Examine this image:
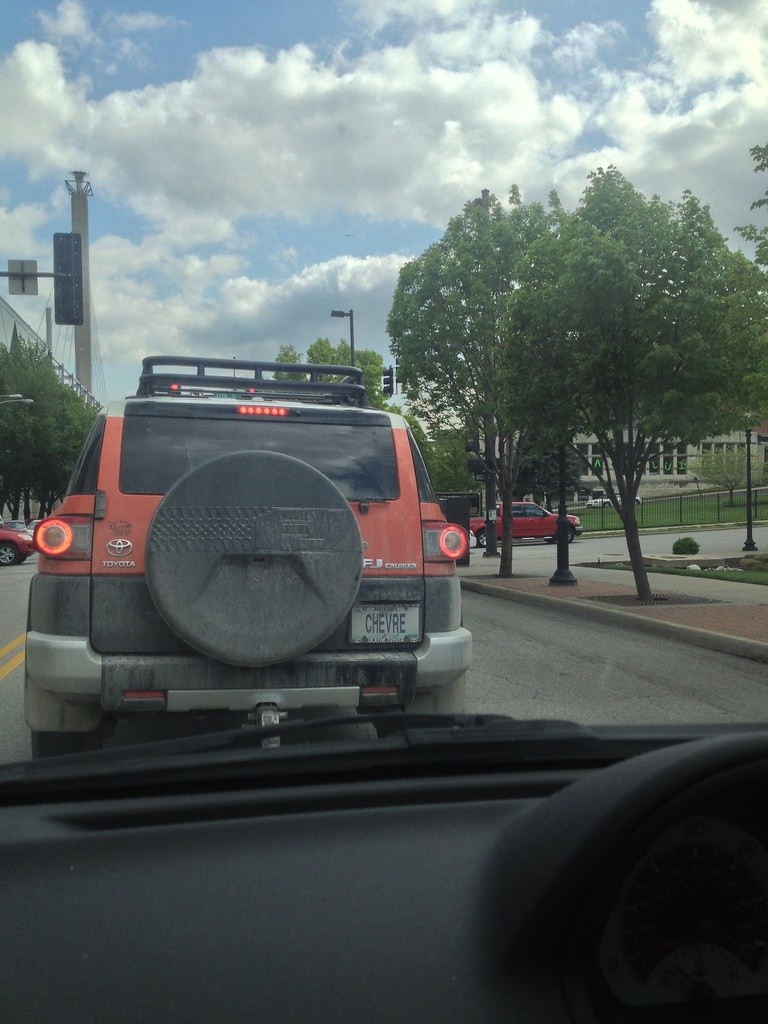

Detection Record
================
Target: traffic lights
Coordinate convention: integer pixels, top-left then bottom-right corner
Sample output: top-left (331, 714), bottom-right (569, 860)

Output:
top-left (396, 356), bottom-right (408, 383)
top-left (382, 365), bottom-right (394, 397)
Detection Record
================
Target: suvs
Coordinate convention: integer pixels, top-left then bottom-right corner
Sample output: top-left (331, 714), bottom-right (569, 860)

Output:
top-left (22, 354), bottom-right (474, 761)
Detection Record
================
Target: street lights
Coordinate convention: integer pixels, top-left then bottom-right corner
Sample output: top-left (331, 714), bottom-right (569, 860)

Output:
top-left (330, 308), bottom-right (355, 367)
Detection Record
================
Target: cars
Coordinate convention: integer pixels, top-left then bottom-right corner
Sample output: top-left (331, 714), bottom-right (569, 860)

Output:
top-left (586, 492), bottom-right (642, 509)
top-left (0, 518), bottom-right (42, 567)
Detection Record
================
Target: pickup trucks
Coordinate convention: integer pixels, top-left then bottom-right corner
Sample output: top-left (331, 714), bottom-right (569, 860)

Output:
top-left (469, 500), bottom-right (582, 548)
